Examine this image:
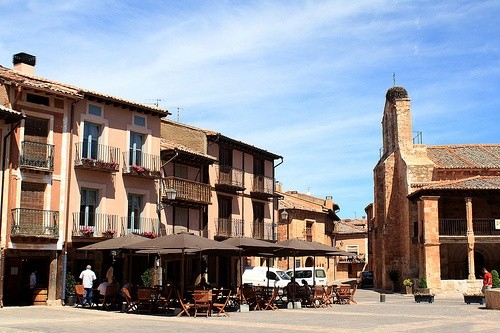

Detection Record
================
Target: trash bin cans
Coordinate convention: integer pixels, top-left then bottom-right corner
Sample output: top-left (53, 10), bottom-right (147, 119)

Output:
top-left (362, 271), bottom-right (374, 288)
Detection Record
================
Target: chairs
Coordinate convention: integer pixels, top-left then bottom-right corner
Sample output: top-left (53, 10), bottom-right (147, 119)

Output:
top-left (76, 284), bottom-right (358, 318)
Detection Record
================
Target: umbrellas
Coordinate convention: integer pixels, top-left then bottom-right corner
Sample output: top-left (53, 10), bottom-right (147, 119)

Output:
top-left (123, 229), bottom-right (242, 300)
top-left (289, 246), bottom-right (353, 284)
top-left (199, 236), bottom-right (284, 283)
top-left (76, 233), bottom-right (151, 251)
top-left (271, 238), bottom-right (341, 283)
top-left (135, 246), bottom-right (199, 254)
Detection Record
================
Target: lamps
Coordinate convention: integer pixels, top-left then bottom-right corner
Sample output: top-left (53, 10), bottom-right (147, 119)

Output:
top-left (277, 209), bottom-right (288, 225)
top-left (158, 186), bottom-right (176, 211)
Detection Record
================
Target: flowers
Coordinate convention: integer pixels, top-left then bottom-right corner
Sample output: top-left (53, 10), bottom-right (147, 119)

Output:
top-left (145, 231), bottom-right (157, 239)
top-left (79, 227), bottom-right (94, 233)
top-left (85, 157), bottom-right (117, 169)
top-left (403, 278), bottom-right (413, 286)
top-left (133, 165), bottom-right (161, 176)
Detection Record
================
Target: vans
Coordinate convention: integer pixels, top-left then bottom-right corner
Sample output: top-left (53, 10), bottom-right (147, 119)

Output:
top-left (280, 265), bottom-right (327, 289)
top-left (358, 270), bottom-right (374, 289)
top-left (241, 266), bottom-right (292, 296)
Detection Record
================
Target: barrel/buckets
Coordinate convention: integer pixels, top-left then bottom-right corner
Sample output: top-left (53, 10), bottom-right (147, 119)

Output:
top-left (361, 271), bottom-right (374, 290)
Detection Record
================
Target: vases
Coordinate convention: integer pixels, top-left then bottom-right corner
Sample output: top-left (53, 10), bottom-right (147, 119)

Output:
top-left (406, 286), bottom-right (413, 295)
top-left (130, 167), bottom-right (160, 179)
top-left (82, 230), bottom-right (94, 237)
top-left (82, 159), bottom-right (115, 170)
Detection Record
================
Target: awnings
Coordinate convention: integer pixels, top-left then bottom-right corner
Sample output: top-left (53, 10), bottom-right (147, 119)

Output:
top-left (0, 104), bottom-right (26, 137)
top-left (12, 80), bottom-right (84, 106)
top-left (160, 148), bottom-right (217, 168)
top-left (206, 131), bottom-right (284, 168)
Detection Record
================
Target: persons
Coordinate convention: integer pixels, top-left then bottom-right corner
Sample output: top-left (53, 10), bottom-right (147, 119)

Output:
top-left (481, 267), bottom-right (493, 292)
top-left (119, 279), bottom-right (132, 296)
top-left (79, 265), bottom-right (97, 307)
top-left (302, 280), bottom-right (312, 295)
top-left (95, 277), bottom-right (110, 306)
top-left (286, 277), bottom-right (300, 299)
top-left (111, 276), bottom-right (120, 292)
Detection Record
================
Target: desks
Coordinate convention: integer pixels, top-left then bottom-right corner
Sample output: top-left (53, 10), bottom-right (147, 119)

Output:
top-left (192, 293), bottom-right (216, 316)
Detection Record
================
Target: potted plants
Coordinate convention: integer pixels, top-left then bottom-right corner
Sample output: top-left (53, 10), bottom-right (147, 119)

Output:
top-left (385, 267), bottom-right (402, 292)
top-left (65, 272), bottom-right (81, 305)
top-left (418, 278), bottom-right (431, 294)
top-left (137, 268), bottom-right (156, 303)
top-left (461, 290), bottom-right (485, 305)
top-left (414, 293), bottom-right (435, 304)
